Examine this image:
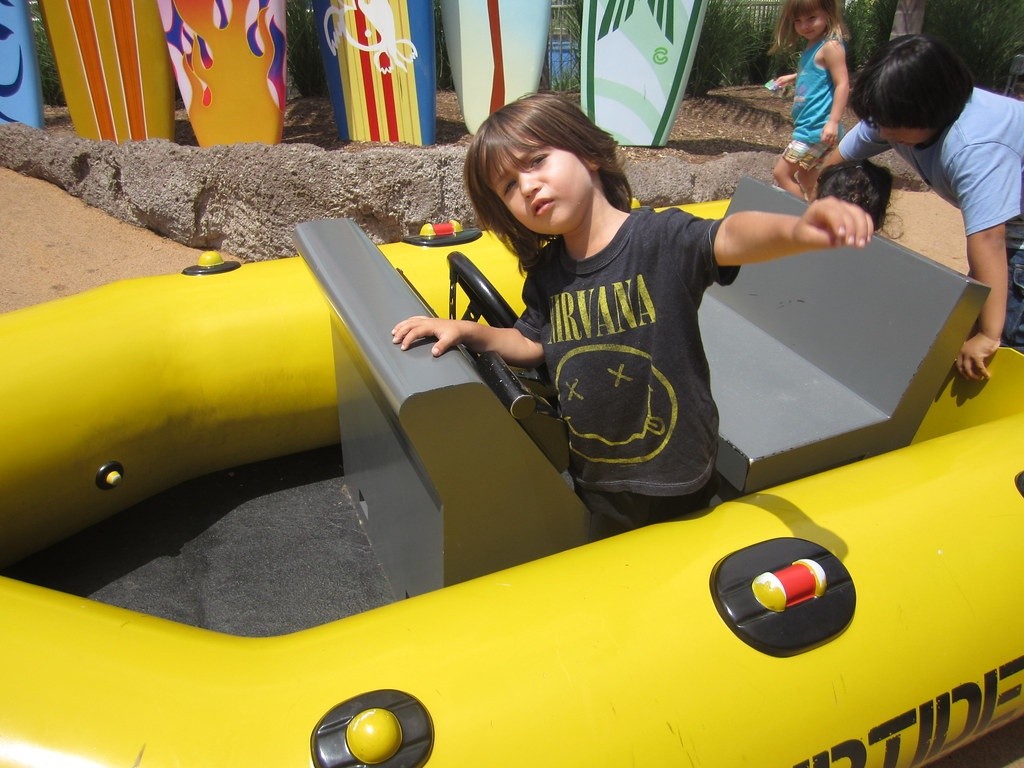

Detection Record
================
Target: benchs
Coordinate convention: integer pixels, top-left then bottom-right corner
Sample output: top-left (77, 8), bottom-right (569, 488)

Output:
top-left (698, 175), bottom-right (991, 503)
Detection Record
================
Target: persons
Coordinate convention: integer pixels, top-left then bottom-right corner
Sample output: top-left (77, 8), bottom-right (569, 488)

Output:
top-left (390, 84), bottom-right (873, 527)
top-left (806, 34), bottom-right (1024, 383)
top-left (772, 0), bottom-right (849, 198)
top-left (816, 158), bottom-right (893, 233)
